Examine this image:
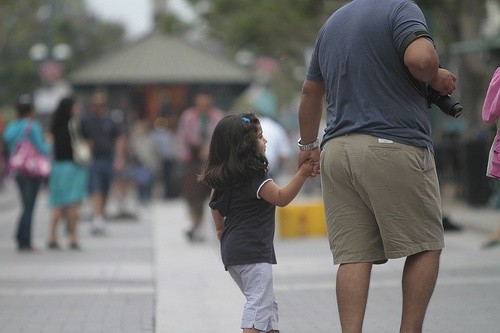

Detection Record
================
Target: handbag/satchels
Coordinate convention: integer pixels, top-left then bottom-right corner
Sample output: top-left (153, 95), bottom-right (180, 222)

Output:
top-left (8, 139), bottom-right (50, 179)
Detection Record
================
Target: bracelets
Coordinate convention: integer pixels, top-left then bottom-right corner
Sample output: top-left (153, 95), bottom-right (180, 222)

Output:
top-left (297, 137), bottom-right (320, 150)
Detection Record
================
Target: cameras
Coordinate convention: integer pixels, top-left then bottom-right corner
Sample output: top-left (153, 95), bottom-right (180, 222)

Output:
top-left (427, 84), bottom-right (464, 118)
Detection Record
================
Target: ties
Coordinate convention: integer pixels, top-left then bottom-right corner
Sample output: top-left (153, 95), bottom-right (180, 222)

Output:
top-left (199, 114), bottom-right (208, 141)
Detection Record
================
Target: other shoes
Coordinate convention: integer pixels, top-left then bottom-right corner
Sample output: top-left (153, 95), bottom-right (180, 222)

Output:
top-left (17, 245), bottom-right (39, 255)
top-left (442, 217), bottom-right (462, 231)
top-left (90, 222), bottom-right (107, 236)
top-left (113, 211), bottom-right (138, 221)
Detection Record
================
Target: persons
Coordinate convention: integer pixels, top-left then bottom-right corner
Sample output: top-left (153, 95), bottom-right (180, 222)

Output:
top-left (482, 66), bottom-right (500, 182)
top-left (177, 92), bottom-right (293, 242)
top-left (197, 112), bottom-right (319, 333)
top-left (0, 89), bottom-right (125, 252)
top-left (298, 0), bottom-right (457, 333)
top-left (120, 117), bottom-right (182, 207)
